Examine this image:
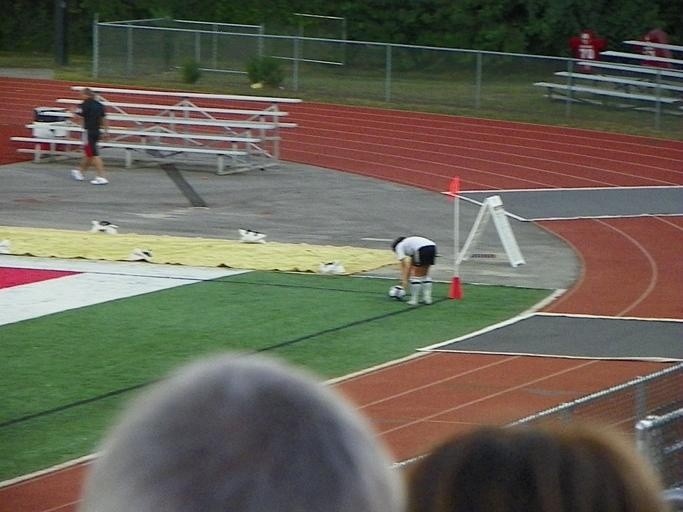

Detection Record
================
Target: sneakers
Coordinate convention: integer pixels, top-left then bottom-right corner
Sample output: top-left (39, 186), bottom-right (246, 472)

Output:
top-left (90, 176), bottom-right (108, 185)
top-left (72, 169), bottom-right (84, 180)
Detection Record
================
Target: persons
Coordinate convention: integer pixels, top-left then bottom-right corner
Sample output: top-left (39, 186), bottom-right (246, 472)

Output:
top-left (392, 236), bottom-right (436, 305)
top-left (71, 88), bottom-right (108, 185)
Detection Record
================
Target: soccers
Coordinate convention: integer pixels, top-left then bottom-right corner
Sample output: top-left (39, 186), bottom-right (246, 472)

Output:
top-left (389, 284), bottom-right (407, 301)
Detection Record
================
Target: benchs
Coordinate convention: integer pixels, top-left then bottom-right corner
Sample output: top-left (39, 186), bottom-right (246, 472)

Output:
top-left (533, 40), bottom-right (683, 128)
top-left (11, 86), bottom-right (302, 175)
